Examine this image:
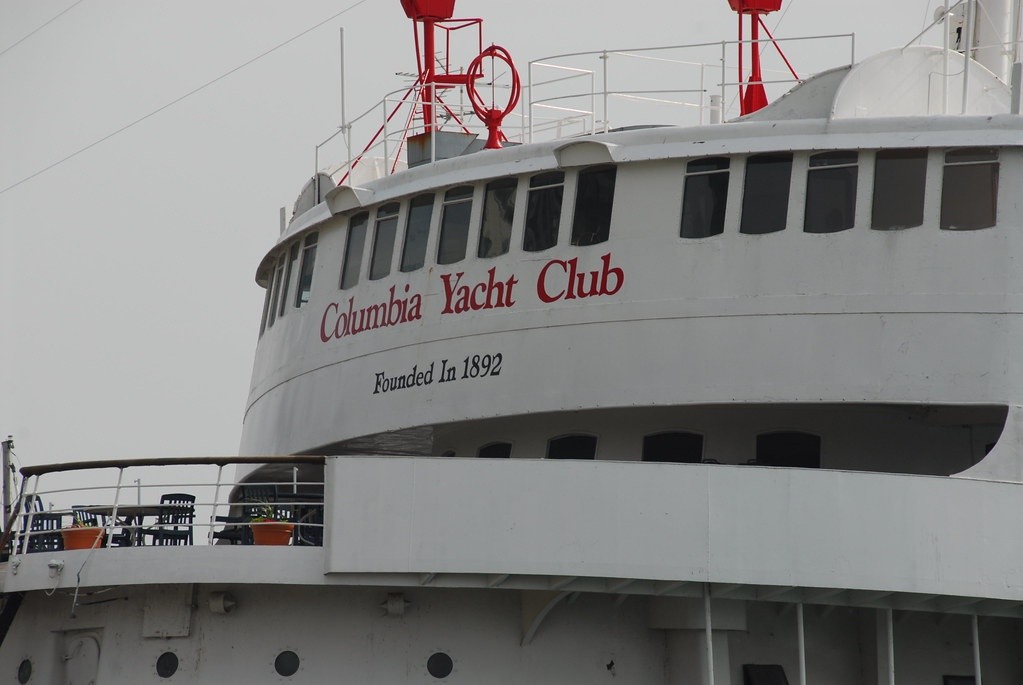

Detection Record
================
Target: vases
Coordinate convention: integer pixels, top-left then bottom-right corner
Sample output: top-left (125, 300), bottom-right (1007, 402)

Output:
top-left (251, 524), bottom-right (295, 545)
top-left (62, 526), bottom-right (105, 550)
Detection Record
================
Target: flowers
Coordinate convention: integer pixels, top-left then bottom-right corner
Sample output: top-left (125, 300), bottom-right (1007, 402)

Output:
top-left (247, 498), bottom-right (289, 524)
top-left (61, 516), bottom-right (93, 528)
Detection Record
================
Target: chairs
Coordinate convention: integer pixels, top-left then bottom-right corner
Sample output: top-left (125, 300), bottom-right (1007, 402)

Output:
top-left (71, 505), bottom-right (98, 528)
top-left (141, 493), bottom-right (196, 546)
top-left (85, 503), bottom-right (177, 547)
top-left (102, 514), bottom-right (135, 547)
top-left (25, 494), bottom-right (44, 513)
top-left (208, 501), bottom-right (254, 545)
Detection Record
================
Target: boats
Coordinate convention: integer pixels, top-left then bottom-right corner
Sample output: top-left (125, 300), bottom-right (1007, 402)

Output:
top-left (1, 0), bottom-right (1023, 685)
